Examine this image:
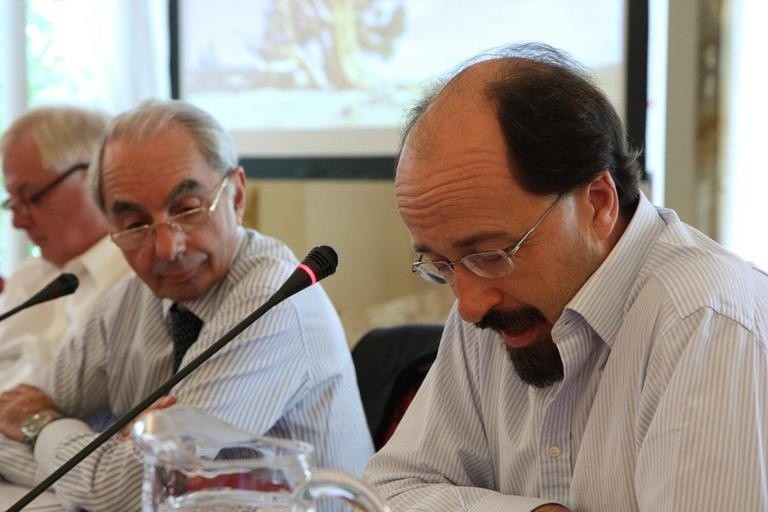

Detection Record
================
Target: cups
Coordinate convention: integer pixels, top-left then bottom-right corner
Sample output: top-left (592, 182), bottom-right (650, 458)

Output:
top-left (131, 404), bottom-right (391, 512)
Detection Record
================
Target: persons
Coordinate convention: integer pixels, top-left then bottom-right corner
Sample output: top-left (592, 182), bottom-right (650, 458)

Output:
top-left (1, 97), bottom-right (377, 511)
top-left (309, 41), bottom-right (768, 512)
top-left (0, 103), bottom-right (138, 396)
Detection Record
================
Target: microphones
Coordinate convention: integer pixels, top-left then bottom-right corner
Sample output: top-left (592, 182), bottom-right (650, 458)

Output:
top-left (4, 244), bottom-right (338, 512)
top-left (0, 273), bottom-right (78, 321)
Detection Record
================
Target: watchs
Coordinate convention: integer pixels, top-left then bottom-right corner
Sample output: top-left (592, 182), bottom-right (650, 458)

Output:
top-left (21, 407), bottom-right (63, 450)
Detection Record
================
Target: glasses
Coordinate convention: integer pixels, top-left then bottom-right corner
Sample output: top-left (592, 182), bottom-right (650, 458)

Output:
top-left (0, 161), bottom-right (89, 216)
top-left (411, 189), bottom-right (567, 285)
top-left (108, 173), bottom-right (229, 252)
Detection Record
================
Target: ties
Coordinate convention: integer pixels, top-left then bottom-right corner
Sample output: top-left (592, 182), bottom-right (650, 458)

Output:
top-left (165, 305), bottom-right (205, 397)
